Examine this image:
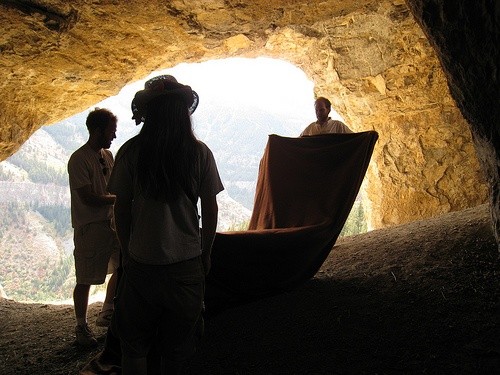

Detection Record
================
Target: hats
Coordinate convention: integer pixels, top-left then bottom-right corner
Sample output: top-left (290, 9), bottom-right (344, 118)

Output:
top-left (131, 76), bottom-right (199, 126)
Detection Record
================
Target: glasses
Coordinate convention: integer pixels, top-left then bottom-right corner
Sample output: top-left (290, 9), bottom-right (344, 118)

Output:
top-left (99, 158), bottom-right (107, 176)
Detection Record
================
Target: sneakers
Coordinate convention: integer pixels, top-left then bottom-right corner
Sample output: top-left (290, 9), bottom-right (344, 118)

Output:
top-left (95, 309), bottom-right (114, 326)
top-left (75, 323), bottom-right (98, 347)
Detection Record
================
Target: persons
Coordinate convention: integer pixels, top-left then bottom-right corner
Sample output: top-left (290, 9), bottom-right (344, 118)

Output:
top-left (67, 105), bottom-right (130, 351)
top-left (110, 73), bottom-right (225, 372)
top-left (289, 97), bottom-right (355, 138)
